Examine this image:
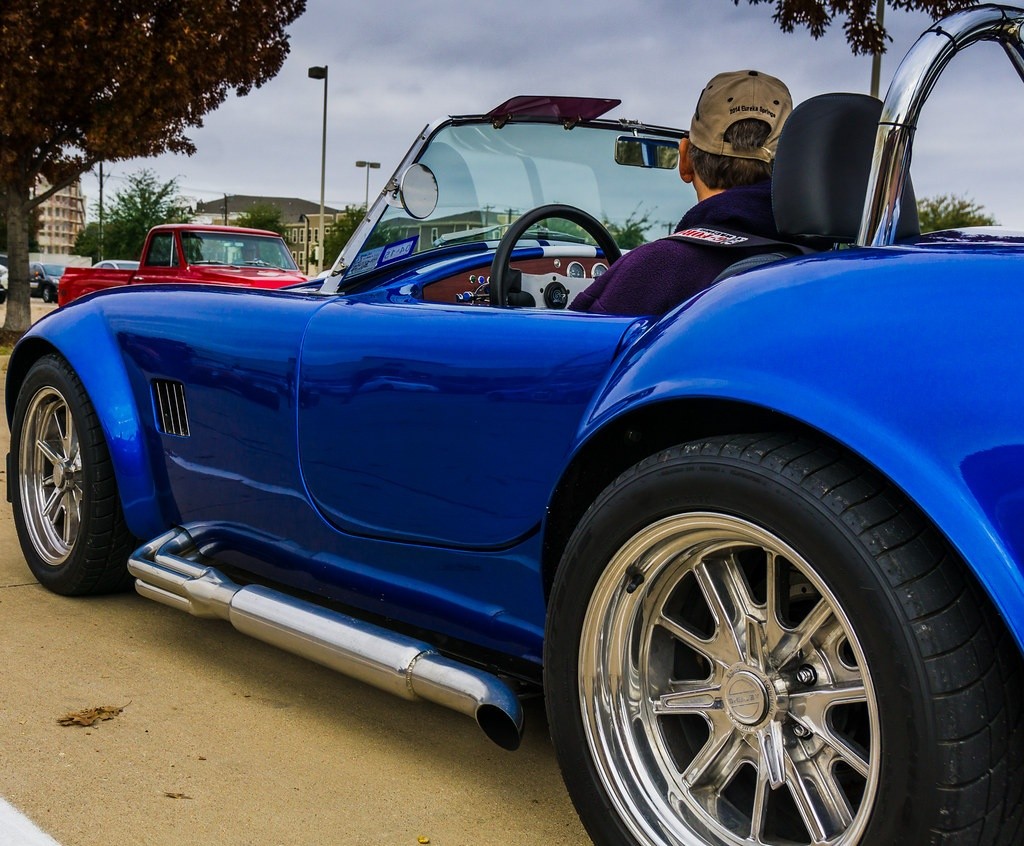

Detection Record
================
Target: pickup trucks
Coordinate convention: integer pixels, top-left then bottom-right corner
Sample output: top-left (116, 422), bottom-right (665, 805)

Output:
top-left (57, 224), bottom-right (309, 306)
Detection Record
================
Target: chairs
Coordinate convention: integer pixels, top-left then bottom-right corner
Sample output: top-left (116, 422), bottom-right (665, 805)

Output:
top-left (709, 93), bottom-right (919, 285)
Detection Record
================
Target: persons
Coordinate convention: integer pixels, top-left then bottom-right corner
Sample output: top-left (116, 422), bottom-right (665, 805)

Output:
top-left (235, 242), bottom-right (264, 265)
top-left (565, 69), bottom-right (793, 318)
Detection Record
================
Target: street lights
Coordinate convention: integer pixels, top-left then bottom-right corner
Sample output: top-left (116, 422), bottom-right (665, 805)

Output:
top-left (305, 64), bottom-right (332, 272)
top-left (354, 160), bottom-right (383, 212)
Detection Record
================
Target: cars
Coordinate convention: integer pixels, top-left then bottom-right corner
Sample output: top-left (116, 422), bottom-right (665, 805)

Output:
top-left (0, 254), bottom-right (42, 303)
top-left (5, 8), bottom-right (1023, 846)
top-left (91, 260), bottom-right (141, 270)
top-left (33, 263), bottom-right (72, 302)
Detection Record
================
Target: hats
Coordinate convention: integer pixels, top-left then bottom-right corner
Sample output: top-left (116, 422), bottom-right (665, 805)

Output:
top-left (689, 69), bottom-right (793, 162)
